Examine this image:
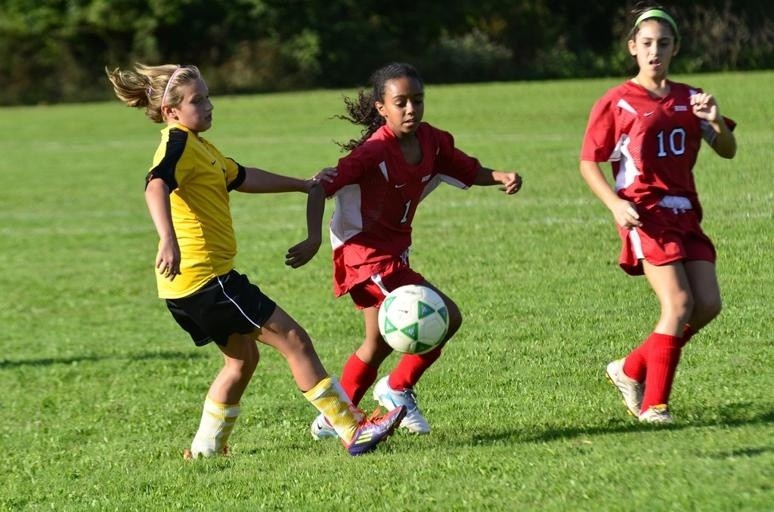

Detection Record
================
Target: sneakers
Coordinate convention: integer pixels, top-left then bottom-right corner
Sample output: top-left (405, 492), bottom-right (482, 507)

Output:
top-left (310, 374), bottom-right (430, 456)
top-left (184, 444), bottom-right (227, 460)
top-left (605, 358), bottom-right (676, 426)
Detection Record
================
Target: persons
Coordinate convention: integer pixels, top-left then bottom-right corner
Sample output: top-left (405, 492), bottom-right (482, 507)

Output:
top-left (105, 59), bottom-right (406, 463)
top-left (579, 0), bottom-right (736, 424)
top-left (285, 63), bottom-right (522, 441)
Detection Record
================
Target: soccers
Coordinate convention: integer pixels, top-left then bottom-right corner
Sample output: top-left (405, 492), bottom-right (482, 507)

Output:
top-left (377, 285), bottom-right (449, 354)
